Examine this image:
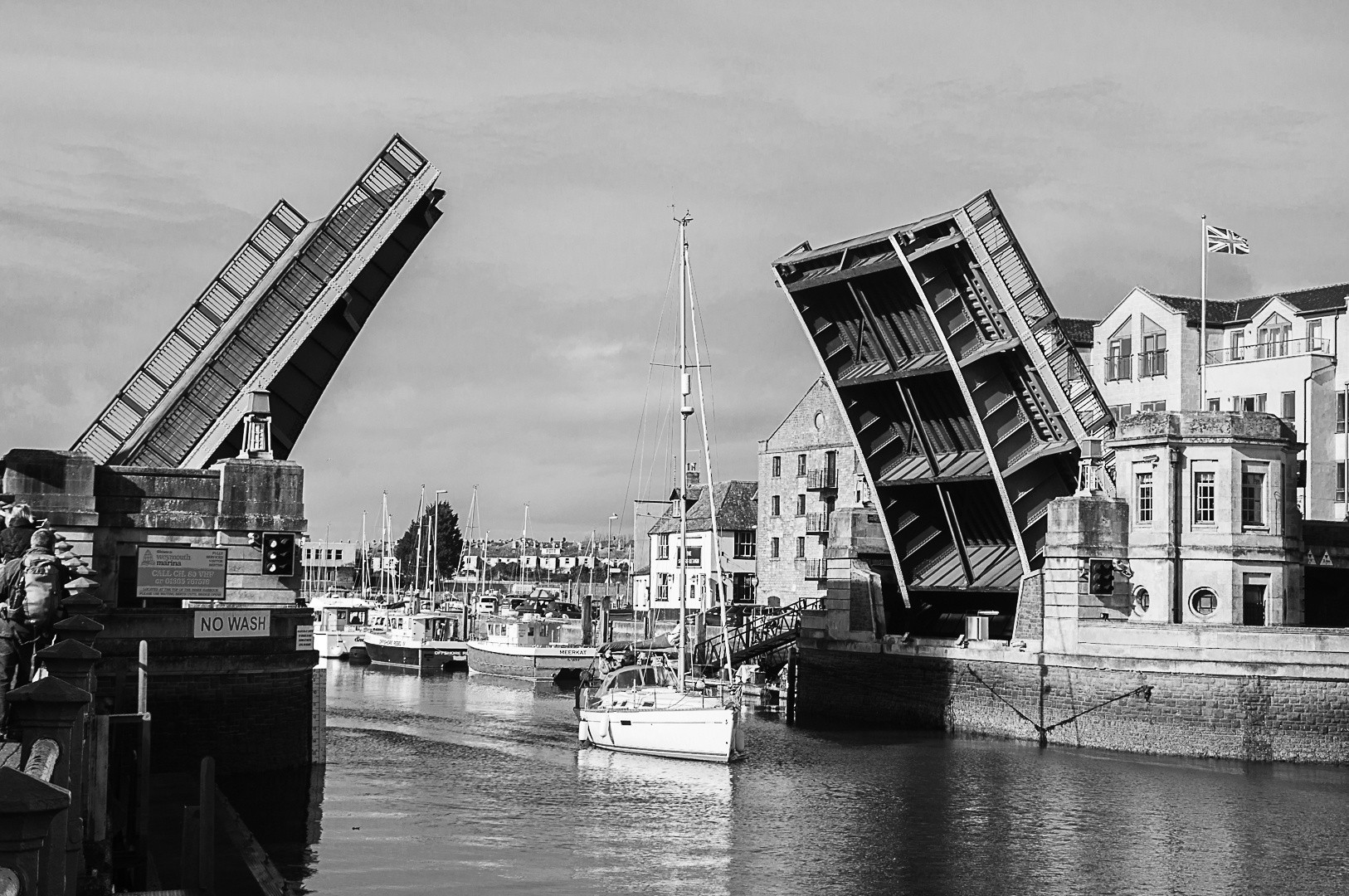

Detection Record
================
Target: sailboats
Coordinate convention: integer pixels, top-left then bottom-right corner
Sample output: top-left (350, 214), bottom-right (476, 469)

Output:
top-left (572, 186), bottom-right (748, 766)
top-left (300, 483), bottom-right (798, 699)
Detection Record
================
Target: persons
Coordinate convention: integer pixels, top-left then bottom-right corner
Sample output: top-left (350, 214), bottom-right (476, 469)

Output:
top-left (0, 504), bottom-right (36, 565)
top-left (0, 528), bottom-right (68, 722)
top-left (620, 648), bottom-right (637, 689)
top-left (599, 650), bottom-right (620, 690)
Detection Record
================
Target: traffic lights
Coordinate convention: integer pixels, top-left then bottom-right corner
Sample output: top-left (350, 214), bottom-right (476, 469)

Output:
top-left (260, 533), bottom-right (296, 577)
top-left (1088, 557), bottom-right (1115, 596)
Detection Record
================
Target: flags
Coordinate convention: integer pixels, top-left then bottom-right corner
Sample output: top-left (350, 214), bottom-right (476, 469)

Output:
top-left (1206, 225), bottom-right (1250, 254)
